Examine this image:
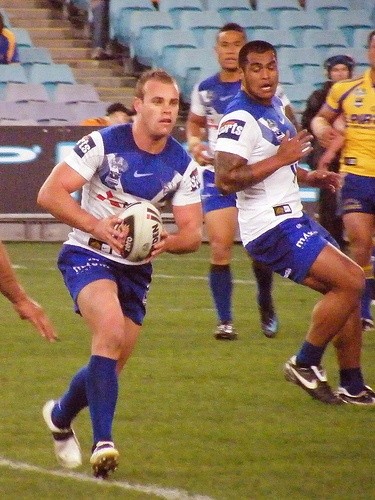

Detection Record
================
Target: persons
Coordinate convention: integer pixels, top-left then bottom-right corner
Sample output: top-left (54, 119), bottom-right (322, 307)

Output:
top-left (47, 0), bottom-right (375, 408)
top-left (1, 245), bottom-right (62, 342)
top-left (36, 72), bottom-right (203, 477)
top-left (0, 13), bottom-right (20, 64)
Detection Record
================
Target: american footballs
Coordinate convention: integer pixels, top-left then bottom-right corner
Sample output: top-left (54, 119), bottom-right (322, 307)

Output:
top-left (112, 201), bottom-right (163, 264)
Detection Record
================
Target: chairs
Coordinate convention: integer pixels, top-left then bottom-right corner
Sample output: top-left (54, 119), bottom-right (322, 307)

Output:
top-left (0, 0), bottom-right (375, 126)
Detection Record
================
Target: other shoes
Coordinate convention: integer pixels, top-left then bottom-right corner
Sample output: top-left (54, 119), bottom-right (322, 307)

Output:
top-left (360, 317), bottom-right (375, 331)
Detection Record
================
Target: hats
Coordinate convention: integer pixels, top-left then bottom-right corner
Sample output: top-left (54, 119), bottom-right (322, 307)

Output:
top-left (327, 56), bottom-right (351, 74)
top-left (106, 102), bottom-right (136, 116)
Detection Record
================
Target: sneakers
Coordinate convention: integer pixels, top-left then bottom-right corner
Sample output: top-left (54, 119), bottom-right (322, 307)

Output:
top-left (215, 321), bottom-right (234, 339)
top-left (42, 400), bottom-right (82, 468)
top-left (284, 355), bottom-right (345, 406)
top-left (336, 384), bottom-right (375, 405)
top-left (90, 441), bottom-right (119, 478)
top-left (257, 294), bottom-right (278, 338)
